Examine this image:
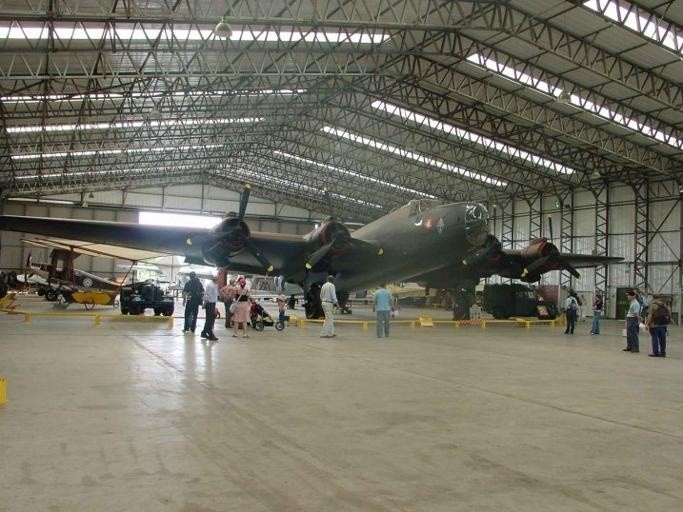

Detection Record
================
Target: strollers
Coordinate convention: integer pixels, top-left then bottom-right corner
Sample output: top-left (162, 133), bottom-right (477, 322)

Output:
top-left (254, 304), bottom-right (284, 331)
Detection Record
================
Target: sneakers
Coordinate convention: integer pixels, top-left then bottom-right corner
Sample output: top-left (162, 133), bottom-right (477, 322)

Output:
top-left (243, 335), bottom-right (250, 338)
top-left (648, 353), bottom-right (659, 357)
top-left (591, 333), bottom-right (598, 335)
top-left (232, 334), bottom-right (238, 337)
top-left (659, 353), bottom-right (665, 357)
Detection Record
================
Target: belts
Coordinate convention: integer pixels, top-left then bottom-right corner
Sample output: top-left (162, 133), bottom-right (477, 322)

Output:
top-left (322, 301), bottom-right (332, 303)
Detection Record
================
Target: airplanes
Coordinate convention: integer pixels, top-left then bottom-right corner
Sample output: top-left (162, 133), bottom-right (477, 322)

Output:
top-left (0, 182), bottom-right (628, 332)
top-left (16, 252), bottom-right (123, 308)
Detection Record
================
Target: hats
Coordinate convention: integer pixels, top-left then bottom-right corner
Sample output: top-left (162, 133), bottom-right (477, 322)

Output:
top-left (239, 279), bottom-right (245, 286)
top-left (276, 294), bottom-right (286, 302)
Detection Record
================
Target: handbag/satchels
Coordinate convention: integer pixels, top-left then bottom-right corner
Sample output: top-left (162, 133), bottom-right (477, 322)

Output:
top-left (228, 304), bottom-right (237, 313)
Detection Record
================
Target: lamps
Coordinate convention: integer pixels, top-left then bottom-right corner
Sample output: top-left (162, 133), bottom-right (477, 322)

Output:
top-left (0, 0), bottom-right (683, 211)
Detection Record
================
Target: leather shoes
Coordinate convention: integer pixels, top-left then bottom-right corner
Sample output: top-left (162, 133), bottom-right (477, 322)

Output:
top-left (201, 332), bottom-right (209, 339)
top-left (320, 333), bottom-right (335, 338)
top-left (623, 349), bottom-right (631, 351)
top-left (209, 334), bottom-right (218, 340)
top-left (630, 350), bottom-right (639, 353)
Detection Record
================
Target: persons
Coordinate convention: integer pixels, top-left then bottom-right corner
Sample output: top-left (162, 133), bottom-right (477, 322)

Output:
top-left (564, 288), bottom-right (587, 336)
top-left (200, 275), bottom-right (220, 341)
top-left (622, 291), bottom-right (638, 353)
top-left (372, 281), bottom-right (396, 339)
top-left (229, 279), bottom-right (253, 338)
top-left (645, 293), bottom-right (670, 357)
top-left (588, 294), bottom-right (602, 334)
top-left (219, 278), bottom-right (236, 328)
top-left (277, 294), bottom-right (286, 324)
top-left (181, 270), bottom-right (203, 335)
top-left (318, 275), bottom-right (340, 337)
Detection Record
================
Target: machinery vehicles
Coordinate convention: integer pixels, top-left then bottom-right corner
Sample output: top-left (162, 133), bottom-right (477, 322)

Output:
top-left (118, 279), bottom-right (174, 316)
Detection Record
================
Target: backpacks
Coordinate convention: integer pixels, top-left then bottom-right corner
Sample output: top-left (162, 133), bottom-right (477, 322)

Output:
top-left (567, 298), bottom-right (576, 311)
top-left (652, 303), bottom-right (669, 325)
